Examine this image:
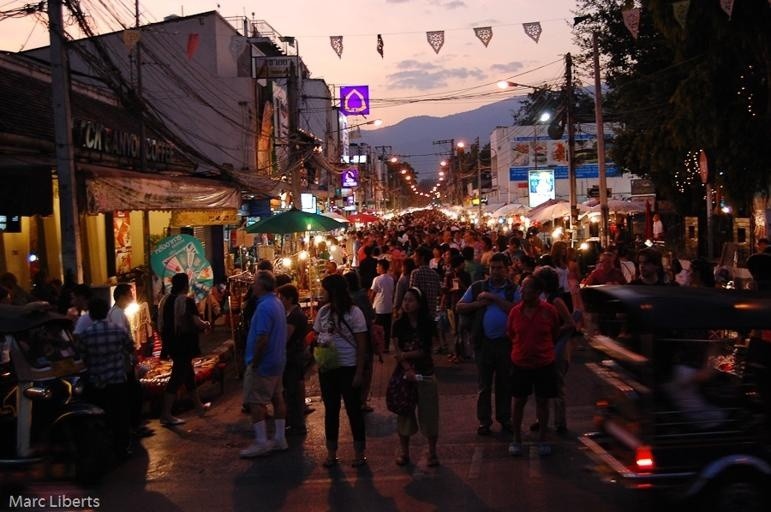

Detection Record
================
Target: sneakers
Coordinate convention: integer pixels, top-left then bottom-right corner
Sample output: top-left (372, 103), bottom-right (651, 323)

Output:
top-left (427, 456), bottom-right (440, 466)
top-left (239, 441), bottom-right (273, 458)
top-left (395, 457), bottom-right (409, 465)
top-left (508, 443), bottom-right (520, 455)
top-left (270, 438), bottom-right (289, 451)
top-left (478, 425), bottom-right (494, 434)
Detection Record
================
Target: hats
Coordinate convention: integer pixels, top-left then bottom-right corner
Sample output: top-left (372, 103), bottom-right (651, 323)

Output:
top-left (0, 301), bottom-right (73, 332)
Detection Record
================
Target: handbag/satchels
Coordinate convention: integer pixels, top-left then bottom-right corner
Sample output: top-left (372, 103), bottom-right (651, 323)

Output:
top-left (186, 322), bottom-right (207, 353)
top-left (462, 312), bottom-right (483, 354)
top-left (386, 364), bottom-right (418, 416)
top-left (358, 337), bottom-right (372, 371)
top-left (448, 272), bottom-right (460, 292)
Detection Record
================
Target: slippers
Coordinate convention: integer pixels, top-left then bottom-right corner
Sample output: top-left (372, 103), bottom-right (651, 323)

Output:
top-left (160, 416), bottom-right (185, 425)
top-left (351, 459), bottom-right (367, 467)
top-left (196, 402), bottom-right (211, 416)
top-left (323, 458), bottom-right (339, 467)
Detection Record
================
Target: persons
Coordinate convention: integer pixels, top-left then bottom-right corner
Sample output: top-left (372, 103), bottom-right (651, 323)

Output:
top-left (0, 271), bottom-right (95, 336)
top-left (630, 248), bottom-right (681, 284)
top-left (685, 258), bottom-right (715, 290)
top-left (389, 287), bottom-right (441, 466)
top-left (313, 274), bottom-right (368, 470)
top-left (367, 260), bottom-right (394, 354)
top-left (240, 260), bottom-right (273, 413)
top-left (460, 246), bottom-right (484, 359)
top-left (498, 218), bottom-right (553, 303)
top-left (297, 223), bottom-right (377, 281)
top-left (76, 298), bottom-right (135, 458)
top-left (107, 284), bottom-right (154, 437)
top-left (274, 273), bottom-right (316, 414)
top-left (432, 268), bottom-right (450, 354)
top-left (441, 257), bottom-right (471, 363)
top-left (236, 233), bottom-right (297, 268)
top-left (408, 246), bottom-right (441, 348)
top-left (652, 214), bottom-right (665, 244)
top-left (377, 205), bottom-right (496, 267)
top-left (581, 234), bottom-right (643, 292)
top-left (392, 258), bottom-right (414, 315)
top-left (277, 284), bottom-right (308, 437)
top-left (157, 273), bottom-right (212, 423)
top-left (566, 248), bottom-right (587, 335)
top-left (758, 239), bottom-right (771, 256)
top-left (506, 275), bottom-right (560, 455)
top-left (553, 240), bottom-right (574, 313)
top-left (345, 273), bottom-right (374, 412)
top-left (456, 253), bottom-right (520, 436)
top-left (530, 268), bottom-right (575, 436)
top-left (237, 270), bottom-right (289, 457)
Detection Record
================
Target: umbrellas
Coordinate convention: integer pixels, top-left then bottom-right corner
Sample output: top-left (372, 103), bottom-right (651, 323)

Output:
top-left (241, 205), bottom-right (349, 236)
top-left (494, 205), bottom-right (530, 217)
top-left (531, 199), bottom-right (592, 223)
top-left (345, 212), bottom-right (379, 225)
top-left (590, 200), bottom-right (645, 230)
top-left (527, 198), bottom-right (571, 217)
top-left (319, 209), bottom-right (347, 222)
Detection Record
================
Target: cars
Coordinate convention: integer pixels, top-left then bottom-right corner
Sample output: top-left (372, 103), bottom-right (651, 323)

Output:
top-left (570, 325), bottom-right (771, 511)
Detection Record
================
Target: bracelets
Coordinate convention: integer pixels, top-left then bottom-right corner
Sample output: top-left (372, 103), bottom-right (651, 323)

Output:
top-left (400, 351), bottom-right (409, 361)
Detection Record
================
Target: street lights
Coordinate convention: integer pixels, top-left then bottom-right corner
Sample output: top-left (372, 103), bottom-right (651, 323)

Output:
top-left (498, 78), bottom-right (586, 228)
top-left (323, 119), bottom-right (383, 154)
top-left (430, 141), bottom-right (465, 216)
top-left (382, 156), bottom-right (429, 218)
